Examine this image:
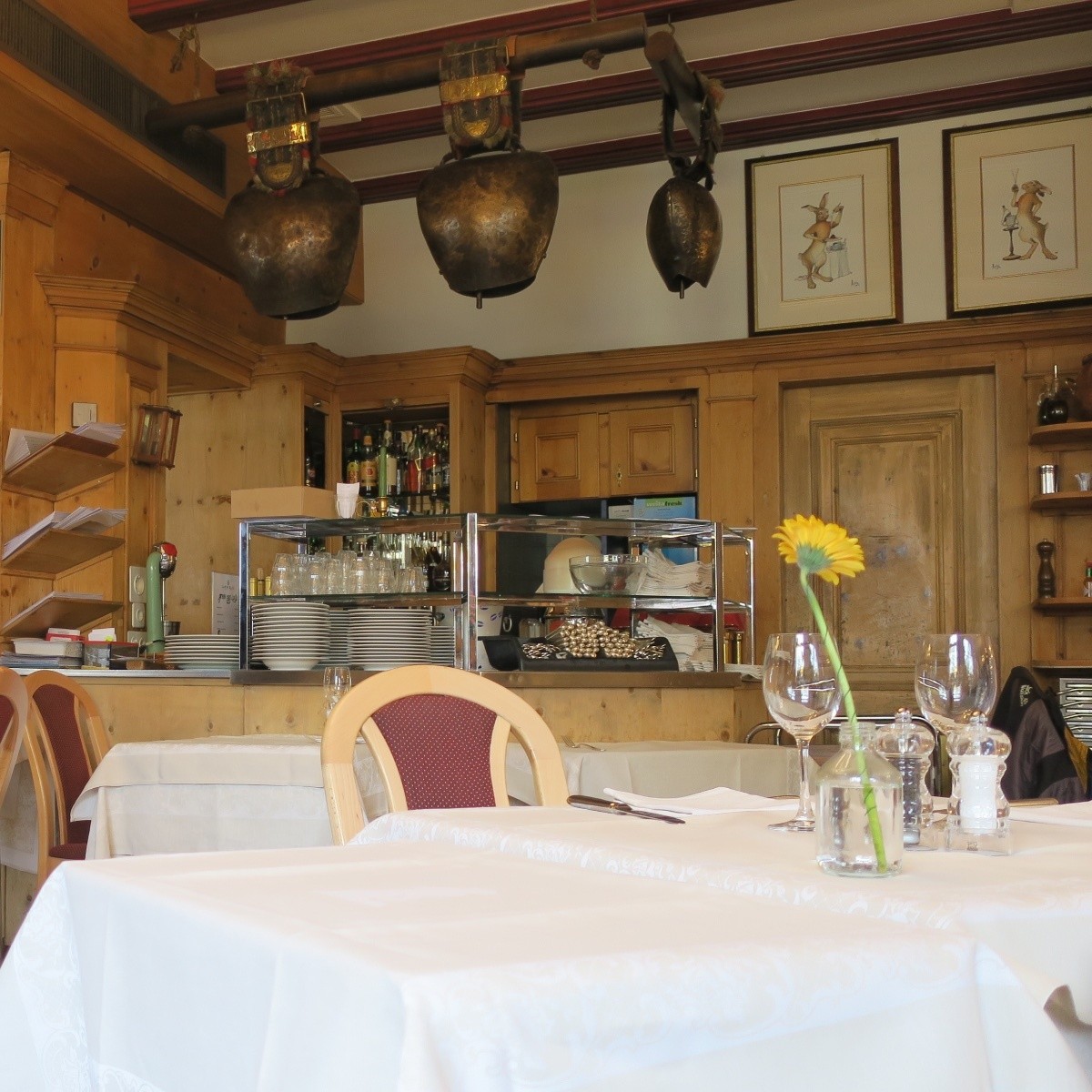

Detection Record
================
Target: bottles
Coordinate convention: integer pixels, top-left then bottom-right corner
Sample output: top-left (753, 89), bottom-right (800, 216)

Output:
top-left (1039, 365), bottom-right (1069, 426)
top-left (345, 420), bottom-right (450, 497)
top-left (1083, 561), bottom-right (1092, 597)
top-left (349, 531), bottom-right (451, 592)
top-left (723, 630), bottom-right (742, 664)
top-left (1040, 464), bottom-right (1059, 495)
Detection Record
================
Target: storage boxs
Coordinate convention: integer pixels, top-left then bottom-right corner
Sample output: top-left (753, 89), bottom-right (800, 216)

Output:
top-left (83, 641), bottom-right (139, 670)
top-left (231, 485), bottom-right (364, 520)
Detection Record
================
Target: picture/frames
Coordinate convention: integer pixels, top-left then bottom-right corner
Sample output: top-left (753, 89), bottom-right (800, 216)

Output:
top-left (942, 107), bottom-right (1092, 320)
top-left (511, 389), bottom-right (699, 504)
top-left (744, 138), bottom-right (905, 338)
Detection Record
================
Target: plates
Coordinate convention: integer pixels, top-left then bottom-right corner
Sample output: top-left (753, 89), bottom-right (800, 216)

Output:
top-left (163, 634), bottom-right (239, 670)
top-left (248, 603), bottom-right (454, 672)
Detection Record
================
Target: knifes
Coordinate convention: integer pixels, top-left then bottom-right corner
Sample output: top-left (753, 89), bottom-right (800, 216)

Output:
top-left (567, 795), bottom-right (685, 824)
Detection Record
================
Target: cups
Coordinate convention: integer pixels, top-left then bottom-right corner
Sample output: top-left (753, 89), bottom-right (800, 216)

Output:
top-left (519, 618), bottom-right (544, 638)
top-left (323, 667), bottom-right (352, 719)
top-left (270, 552), bottom-right (428, 595)
top-left (1074, 472), bottom-right (1092, 491)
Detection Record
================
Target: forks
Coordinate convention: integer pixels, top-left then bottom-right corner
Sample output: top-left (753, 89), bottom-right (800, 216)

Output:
top-left (561, 735), bottom-right (606, 751)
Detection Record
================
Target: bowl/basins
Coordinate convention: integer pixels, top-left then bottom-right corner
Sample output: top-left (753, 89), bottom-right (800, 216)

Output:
top-left (569, 554), bottom-right (648, 595)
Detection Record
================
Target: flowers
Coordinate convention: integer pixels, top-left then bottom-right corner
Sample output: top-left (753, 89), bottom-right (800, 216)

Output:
top-left (772, 513), bottom-right (886, 871)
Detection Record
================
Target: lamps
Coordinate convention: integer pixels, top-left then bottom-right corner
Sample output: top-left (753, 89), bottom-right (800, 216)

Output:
top-left (130, 403), bottom-right (183, 470)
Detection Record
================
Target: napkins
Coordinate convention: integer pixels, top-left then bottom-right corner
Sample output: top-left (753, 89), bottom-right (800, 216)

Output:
top-left (626, 547), bottom-right (713, 597)
top-left (636, 614), bottom-right (764, 679)
top-left (602, 787), bottom-right (815, 818)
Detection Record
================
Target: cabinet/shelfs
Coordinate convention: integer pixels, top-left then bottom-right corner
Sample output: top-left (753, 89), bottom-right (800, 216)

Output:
top-left (239, 512), bottom-right (754, 685)
top-left (0, 446), bottom-right (125, 639)
top-left (1029, 421), bottom-right (1091, 669)
top-left (334, 345), bottom-right (499, 594)
top-left (166, 342), bottom-right (343, 636)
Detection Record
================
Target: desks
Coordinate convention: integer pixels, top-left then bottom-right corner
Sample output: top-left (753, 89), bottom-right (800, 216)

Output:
top-left (70, 734), bottom-right (842, 861)
top-left (0, 802), bottom-right (1092, 1091)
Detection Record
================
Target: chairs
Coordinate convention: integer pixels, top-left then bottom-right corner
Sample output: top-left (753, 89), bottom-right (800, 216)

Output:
top-left (23, 670), bottom-right (111, 899)
top-left (989, 667), bottom-right (1091, 803)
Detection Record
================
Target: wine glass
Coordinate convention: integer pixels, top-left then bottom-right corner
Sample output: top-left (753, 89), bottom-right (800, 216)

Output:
top-left (914, 633), bottom-right (996, 835)
top-left (762, 633), bottom-right (841, 832)
